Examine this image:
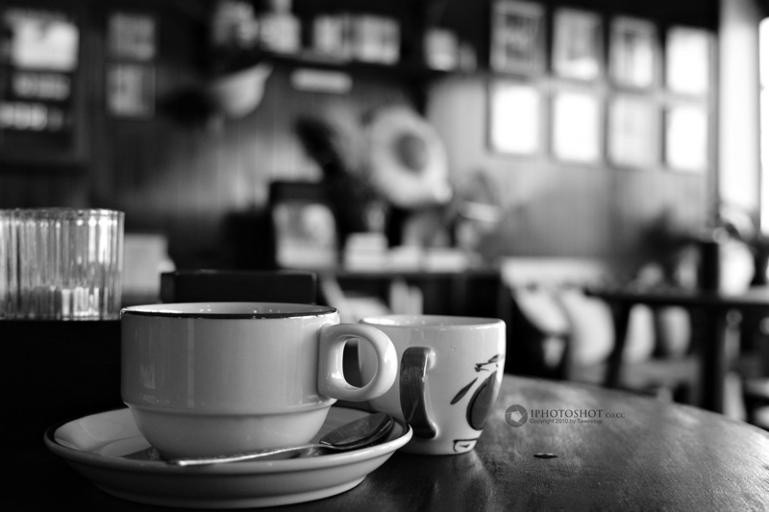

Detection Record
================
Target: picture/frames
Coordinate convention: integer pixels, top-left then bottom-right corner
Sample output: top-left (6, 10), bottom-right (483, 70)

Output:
top-left (485, 1), bottom-right (717, 171)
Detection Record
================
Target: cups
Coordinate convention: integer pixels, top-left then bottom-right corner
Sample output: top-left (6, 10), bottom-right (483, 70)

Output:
top-left (359, 314), bottom-right (507, 454)
top-left (117, 300), bottom-right (399, 459)
top-left (0, 206), bottom-right (134, 318)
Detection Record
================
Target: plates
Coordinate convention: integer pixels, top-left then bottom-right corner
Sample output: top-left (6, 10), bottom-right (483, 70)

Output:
top-left (44, 404), bottom-right (414, 506)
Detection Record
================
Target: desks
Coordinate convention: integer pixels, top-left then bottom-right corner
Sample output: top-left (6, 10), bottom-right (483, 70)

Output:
top-left (580, 278), bottom-right (768, 427)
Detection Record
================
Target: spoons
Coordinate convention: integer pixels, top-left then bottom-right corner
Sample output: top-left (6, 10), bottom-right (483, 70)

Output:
top-left (168, 411), bottom-right (393, 470)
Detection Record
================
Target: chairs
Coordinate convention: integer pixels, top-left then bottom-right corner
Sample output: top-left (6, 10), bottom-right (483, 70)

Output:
top-left (158, 268), bottom-right (322, 306)
